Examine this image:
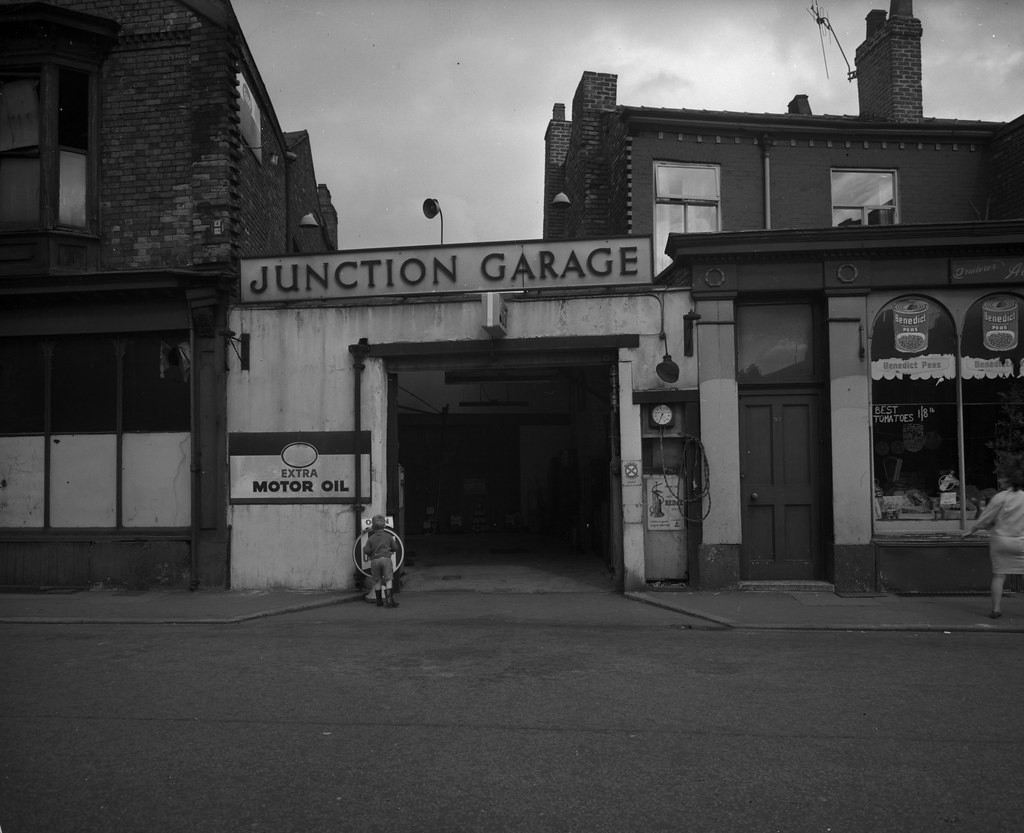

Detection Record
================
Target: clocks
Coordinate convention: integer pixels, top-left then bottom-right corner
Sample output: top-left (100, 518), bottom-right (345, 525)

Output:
top-left (649, 404), bottom-right (675, 428)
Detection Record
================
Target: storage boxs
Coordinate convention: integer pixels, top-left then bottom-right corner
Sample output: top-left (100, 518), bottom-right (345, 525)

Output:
top-left (895, 495), bottom-right (978, 521)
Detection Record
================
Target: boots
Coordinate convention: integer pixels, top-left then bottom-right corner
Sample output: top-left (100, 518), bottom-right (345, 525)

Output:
top-left (375, 590), bottom-right (383, 606)
top-left (384, 588), bottom-right (399, 607)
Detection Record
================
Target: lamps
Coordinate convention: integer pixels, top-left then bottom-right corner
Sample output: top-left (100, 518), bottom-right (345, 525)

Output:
top-left (552, 193), bottom-right (571, 210)
top-left (423, 198), bottom-right (444, 245)
top-left (217, 327), bottom-right (250, 370)
top-left (656, 332), bottom-right (679, 384)
top-left (237, 141), bottom-right (279, 166)
top-left (299, 208), bottom-right (335, 251)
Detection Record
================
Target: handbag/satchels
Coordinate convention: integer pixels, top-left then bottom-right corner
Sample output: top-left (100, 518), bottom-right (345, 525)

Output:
top-left (985, 522), bottom-right (995, 531)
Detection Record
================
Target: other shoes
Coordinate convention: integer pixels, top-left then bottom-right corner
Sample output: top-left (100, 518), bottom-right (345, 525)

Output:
top-left (991, 610), bottom-right (1001, 618)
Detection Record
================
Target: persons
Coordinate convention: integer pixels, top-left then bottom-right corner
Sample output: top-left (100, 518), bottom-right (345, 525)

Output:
top-left (961, 458), bottom-right (1024, 617)
top-left (363, 515), bottom-right (400, 608)
